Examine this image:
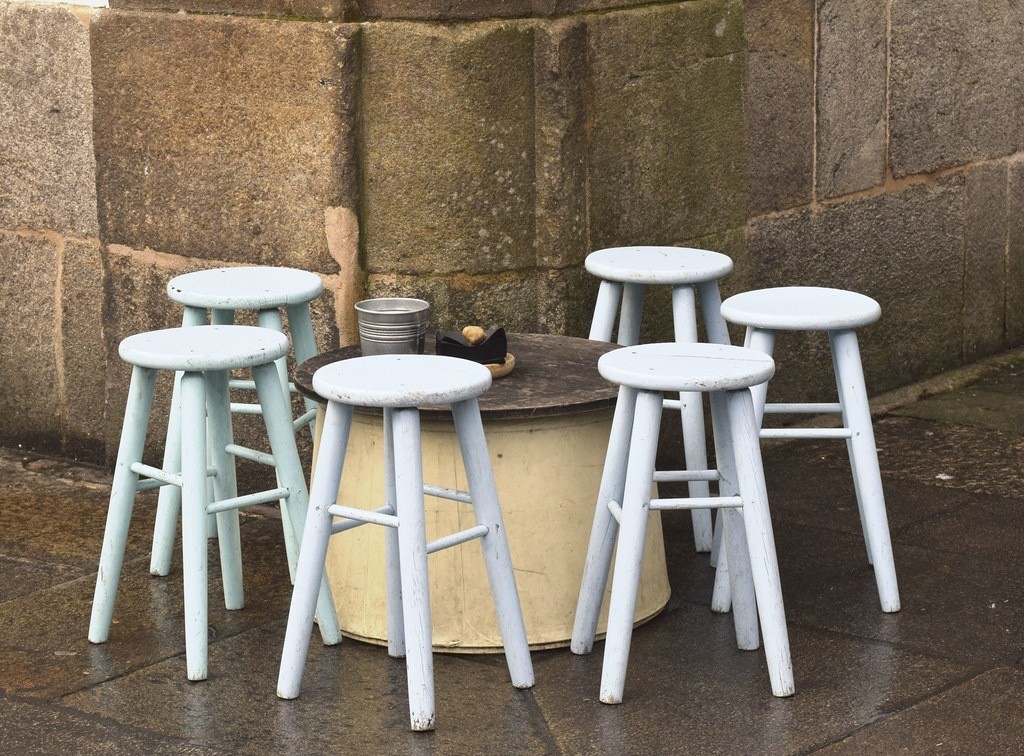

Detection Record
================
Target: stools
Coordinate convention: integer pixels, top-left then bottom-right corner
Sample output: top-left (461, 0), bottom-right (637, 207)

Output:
top-left (278, 353), bottom-right (536, 729)
top-left (585, 245), bottom-right (732, 551)
top-left (569, 342), bottom-right (797, 702)
top-left (709, 285), bottom-right (901, 613)
top-left (150, 266), bottom-right (324, 582)
top-left (83, 325), bottom-right (341, 682)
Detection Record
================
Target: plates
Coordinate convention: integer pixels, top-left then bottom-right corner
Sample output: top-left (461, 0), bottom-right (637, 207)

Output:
top-left (483, 353), bottom-right (517, 378)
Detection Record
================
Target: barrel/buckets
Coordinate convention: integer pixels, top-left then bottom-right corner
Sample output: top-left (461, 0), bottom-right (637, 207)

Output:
top-left (354, 299), bottom-right (429, 356)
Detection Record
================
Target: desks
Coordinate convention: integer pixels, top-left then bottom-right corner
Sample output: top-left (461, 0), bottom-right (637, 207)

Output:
top-left (294, 334), bottom-right (670, 653)
